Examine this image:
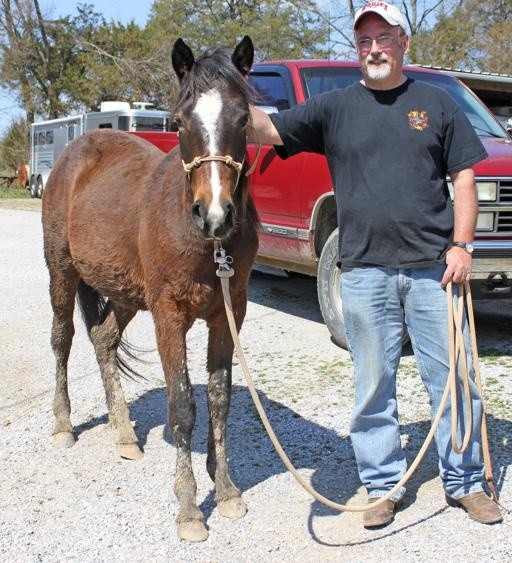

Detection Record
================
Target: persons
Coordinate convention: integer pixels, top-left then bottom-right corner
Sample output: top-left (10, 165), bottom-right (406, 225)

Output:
top-left (249, 2), bottom-right (503, 528)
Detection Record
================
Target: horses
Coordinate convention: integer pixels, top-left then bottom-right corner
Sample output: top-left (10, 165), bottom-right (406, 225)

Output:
top-left (40, 34), bottom-right (266, 542)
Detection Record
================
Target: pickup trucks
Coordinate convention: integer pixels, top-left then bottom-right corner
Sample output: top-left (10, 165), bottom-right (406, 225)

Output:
top-left (124, 58), bottom-right (511, 351)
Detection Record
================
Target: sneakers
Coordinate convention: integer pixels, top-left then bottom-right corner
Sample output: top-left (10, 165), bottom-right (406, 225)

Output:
top-left (362, 496), bottom-right (404, 528)
top-left (445, 491), bottom-right (503, 524)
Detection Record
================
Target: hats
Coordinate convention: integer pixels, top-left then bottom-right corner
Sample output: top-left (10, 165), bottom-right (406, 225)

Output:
top-left (354, 1), bottom-right (412, 40)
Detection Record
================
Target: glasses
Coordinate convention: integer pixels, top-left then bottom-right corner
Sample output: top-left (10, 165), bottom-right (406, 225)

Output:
top-left (355, 35), bottom-right (395, 52)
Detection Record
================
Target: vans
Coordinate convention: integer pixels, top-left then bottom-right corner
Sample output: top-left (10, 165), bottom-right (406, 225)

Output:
top-left (28, 101), bottom-right (180, 198)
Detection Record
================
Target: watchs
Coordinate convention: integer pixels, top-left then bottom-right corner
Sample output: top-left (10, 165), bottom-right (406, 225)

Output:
top-left (452, 242), bottom-right (474, 254)
